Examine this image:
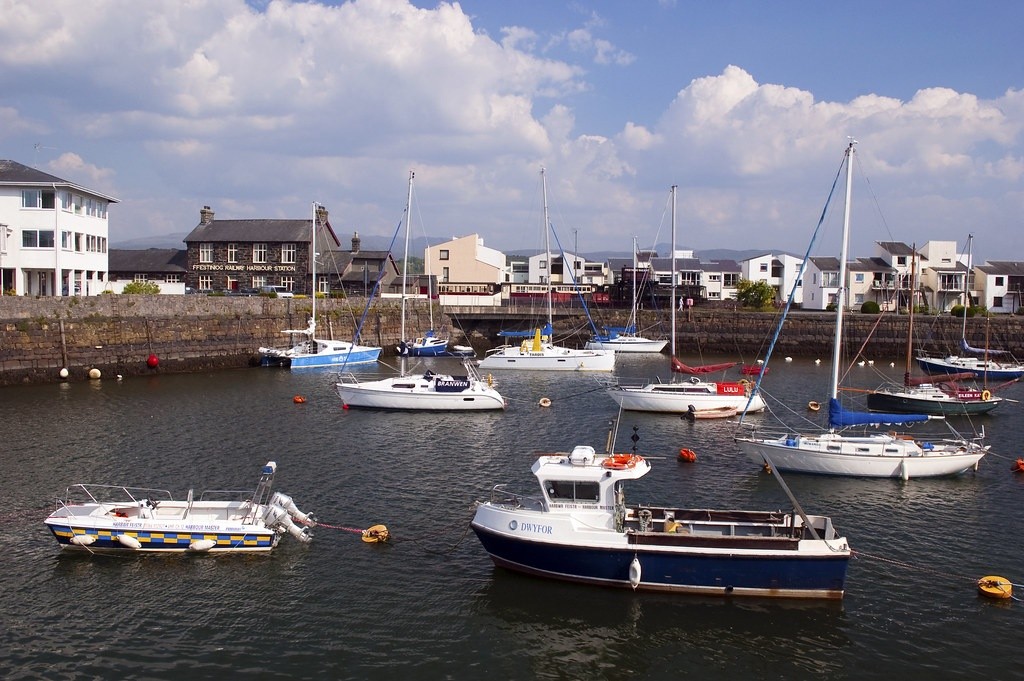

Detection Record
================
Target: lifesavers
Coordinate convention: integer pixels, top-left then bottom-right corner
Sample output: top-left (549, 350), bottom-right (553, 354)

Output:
top-left (602, 453), bottom-right (643, 470)
top-left (539, 397), bottom-right (551, 407)
top-left (362, 525), bottom-right (388, 543)
top-left (981, 390), bottom-right (991, 400)
top-left (739, 378), bottom-right (748, 384)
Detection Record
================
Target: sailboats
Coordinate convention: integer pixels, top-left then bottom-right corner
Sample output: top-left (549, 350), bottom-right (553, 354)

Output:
top-left (391, 245), bottom-right (479, 360)
top-left (913, 233), bottom-right (1024, 382)
top-left (593, 184), bottom-right (768, 416)
top-left (473, 163), bottom-right (616, 374)
top-left (836, 241), bottom-right (1024, 420)
top-left (256, 200), bottom-right (383, 365)
top-left (582, 235), bottom-right (671, 354)
top-left (726, 135), bottom-right (993, 485)
top-left (333, 170), bottom-right (505, 415)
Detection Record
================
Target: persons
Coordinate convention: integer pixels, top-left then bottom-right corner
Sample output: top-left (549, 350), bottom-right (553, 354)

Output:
top-left (678, 296), bottom-right (684, 311)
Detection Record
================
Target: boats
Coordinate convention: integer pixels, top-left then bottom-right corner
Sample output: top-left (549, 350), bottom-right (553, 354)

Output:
top-left (467, 394), bottom-right (851, 603)
top-left (682, 405), bottom-right (739, 420)
top-left (43, 461), bottom-right (318, 556)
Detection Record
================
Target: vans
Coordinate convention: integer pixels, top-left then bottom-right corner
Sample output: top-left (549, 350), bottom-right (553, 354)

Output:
top-left (259, 285), bottom-right (295, 301)
